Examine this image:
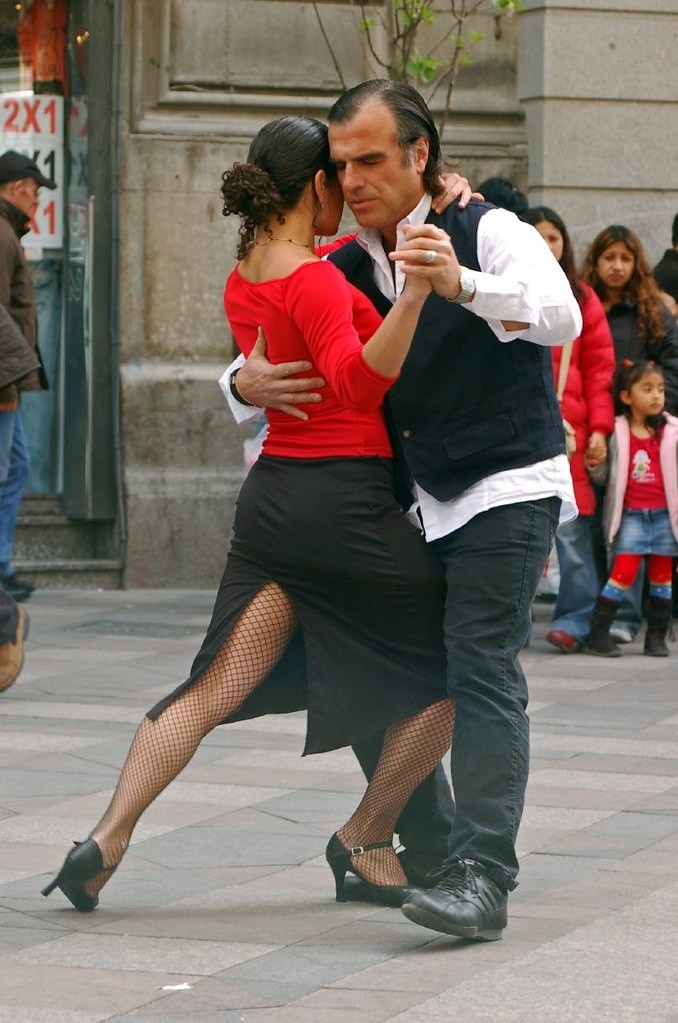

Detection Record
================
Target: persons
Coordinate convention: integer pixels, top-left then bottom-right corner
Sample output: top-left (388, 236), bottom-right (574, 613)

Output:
top-left (41, 115), bottom-right (485, 911)
top-left (220, 80), bottom-right (584, 941)
top-left (479, 178), bottom-right (678, 657)
top-left (0, 150), bottom-right (58, 689)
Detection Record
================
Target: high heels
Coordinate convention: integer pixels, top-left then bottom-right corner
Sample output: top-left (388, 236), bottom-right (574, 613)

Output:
top-left (40, 838), bottom-right (103, 912)
top-left (324, 830), bottom-right (415, 907)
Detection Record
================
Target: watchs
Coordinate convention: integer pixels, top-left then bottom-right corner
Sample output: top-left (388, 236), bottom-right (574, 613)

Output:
top-left (445, 266), bottom-right (475, 304)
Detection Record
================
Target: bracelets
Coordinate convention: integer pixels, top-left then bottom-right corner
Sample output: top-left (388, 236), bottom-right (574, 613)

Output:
top-left (230, 368), bottom-right (253, 405)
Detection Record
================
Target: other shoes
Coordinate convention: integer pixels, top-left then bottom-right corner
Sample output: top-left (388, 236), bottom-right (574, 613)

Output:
top-left (610, 619), bottom-right (635, 642)
top-left (0, 577), bottom-right (36, 601)
top-left (0, 606), bottom-right (29, 691)
top-left (546, 630), bottom-right (581, 654)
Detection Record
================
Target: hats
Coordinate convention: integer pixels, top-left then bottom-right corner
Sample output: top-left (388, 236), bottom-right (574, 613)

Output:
top-left (0, 150), bottom-right (58, 190)
top-left (475, 178), bottom-right (529, 219)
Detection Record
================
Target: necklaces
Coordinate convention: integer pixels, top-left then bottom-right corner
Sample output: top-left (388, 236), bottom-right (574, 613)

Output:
top-left (253, 237), bottom-right (313, 250)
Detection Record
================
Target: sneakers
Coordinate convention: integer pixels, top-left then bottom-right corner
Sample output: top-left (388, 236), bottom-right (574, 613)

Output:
top-left (400, 853), bottom-right (519, 942)
top-left (343, 848), bottom-right (441, 905)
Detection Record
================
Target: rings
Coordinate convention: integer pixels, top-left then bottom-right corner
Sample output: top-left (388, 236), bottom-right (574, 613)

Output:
top-left (426, 251), bottom-right (437, 263)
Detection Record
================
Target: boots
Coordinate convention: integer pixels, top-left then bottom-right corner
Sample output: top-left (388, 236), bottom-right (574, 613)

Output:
top-left (585, 597), bottom-right (623, 656)
top-left (645, 597), bottom-right (669, 657)
